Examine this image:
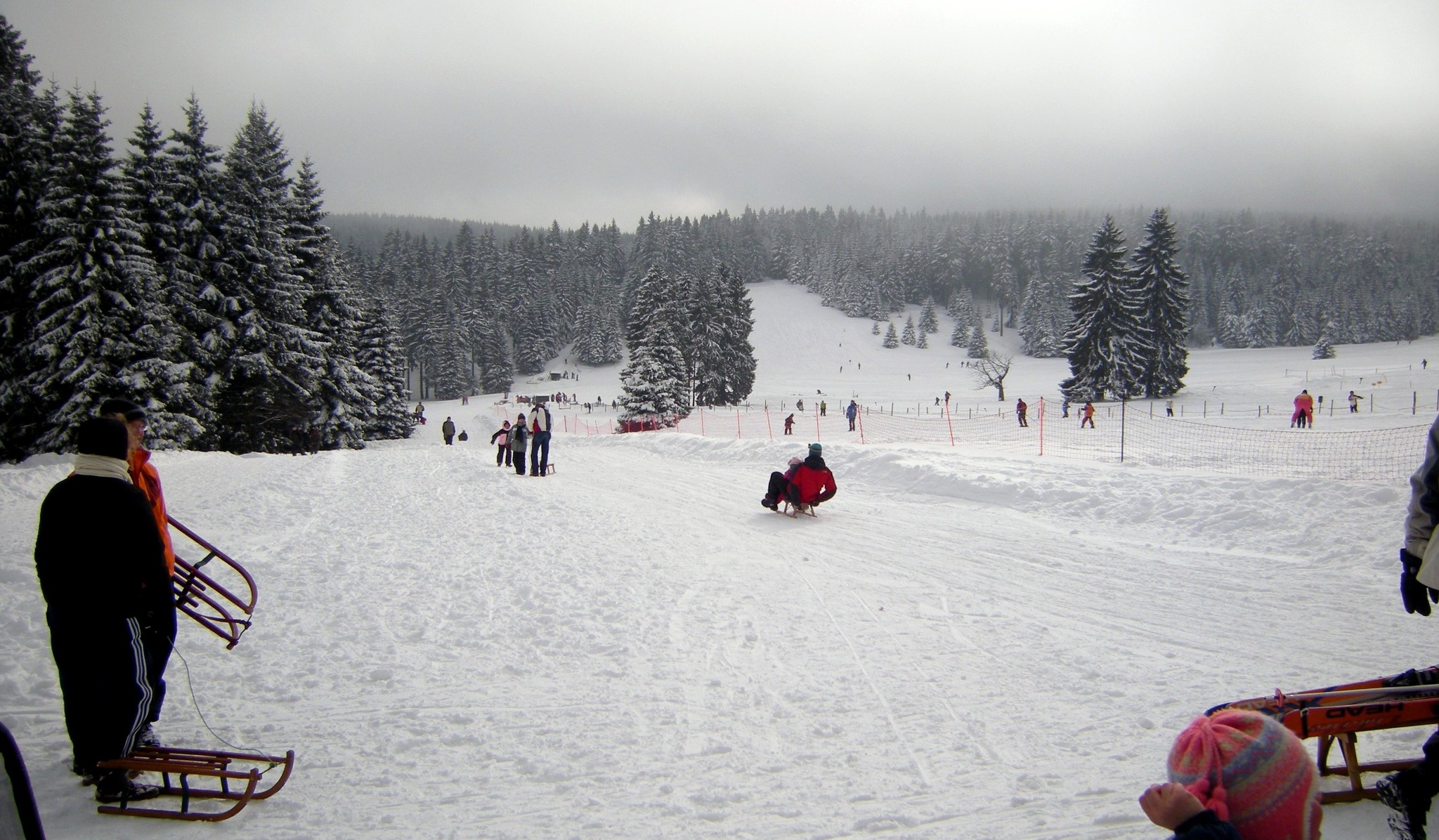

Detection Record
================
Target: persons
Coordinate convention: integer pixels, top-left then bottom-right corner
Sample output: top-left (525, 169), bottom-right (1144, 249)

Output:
top-left (563, 370), bottom-right (579, 381)
top-left (598, 396), bottom-right (601, 406)
top-left (935, 397), bottom-right (939, 406)
top-left (442, 417), bottom-right (468, 445)
top-left (1421, 359), bottom-right (1427, 370)
top-left (945, 391), bottom-right (951, 405)
top-left (1348, 391), bottom-right (1365, 413)
top-left (492, 391), bottom-right (576, 477)
top-left (1080, 401), bottom-right (1095, 428)
top-left (840, 362), bottom-right (861, 373)
top-left (1166, 398), bottom-right (1174, 416)
top-left (946, 360), bottom-right (979, 368)
top-left (462, 395), bottom-right (469, 405)
top-left (846, 400), bottom-right (857, 431)
top-left (1138, 413), bottom-right (1439, 840)
top-left (33, 398), bottom-right (178, 804)
top-left (1016, 398), bottom-right (1028, 427)
top-left (565, 358), bottom-right (567, 364)
top-left (761, 443), bottom-right (837, 511)
top-left (1290, 390), bottom-right (1313, 428)
top-left (411, 402), bottom-right (427, 425)
top-left (584, 403), bottom-right (591, 413)
top-left (797, 399), bottom-right (804, 411)
top-left (1062, 400), bottom-right (1070, 418)
top-left (907, 374), bottom-right (911, 381)
top-left (612, 399), bottom-right (617, 410)
top-left (820, 400), bottom-right (826, 416)
top-left (784, 414), bottom-right (795, 435)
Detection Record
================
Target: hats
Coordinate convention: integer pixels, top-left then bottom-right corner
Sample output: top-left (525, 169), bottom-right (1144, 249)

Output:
top-left (788, 456), bottom-right (804, 465)
top-left (808, 443), bottom-right (822, 457)
top-left (1165, 708), bottom-right (1319, 840)
top-left (100, 399), bottom-right (147, 427)
top-left (518, 413), bottom-right (526, 420)
top-left (535, 403), bottom-right (545, 408)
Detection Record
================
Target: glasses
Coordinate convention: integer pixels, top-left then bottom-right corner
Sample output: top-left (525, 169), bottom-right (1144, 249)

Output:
top-left (126, 423), bottom-right (148, 431)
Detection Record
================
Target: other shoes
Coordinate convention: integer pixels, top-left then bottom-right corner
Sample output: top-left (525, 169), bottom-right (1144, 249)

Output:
top-left (94, 777), bottom-right (159, 803)
top-left (1291, 422), bottom-right (1295, 428)
top-left (530, 473), bottom-right (538, 476)
top-left (1308, 423), bottom-right (1312, 428)
top-left (73, 761), bottom-right (130, 778)
top-left (498, 463), bottom-right (501, 466)
top-left (506, 464), bottom-right (512, 467)
top-left (541, 474), bottom-right (545, 476)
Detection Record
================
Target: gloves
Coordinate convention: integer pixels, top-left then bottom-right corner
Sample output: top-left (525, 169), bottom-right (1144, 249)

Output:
top-left (506, 443), bottom-right (509, 448)
top-left (528, 430), bottom-right (533, 435)
top-left (792, 501), bottom-right (801, 506)
top-left (491, 440), bottom-right (494, 445)
top-left (812, 498), bottom-right (820, 506)
top-left (1400, 548), bottom-right (1439, 617)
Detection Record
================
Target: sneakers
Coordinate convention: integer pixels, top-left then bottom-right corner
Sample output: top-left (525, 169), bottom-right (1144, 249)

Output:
top-left (136, 722), bottom-right (160, 747)
top-left (761, 499), bottom-right (778, 511)
top-left (1376, 768), bottom-right (1432, 840)
